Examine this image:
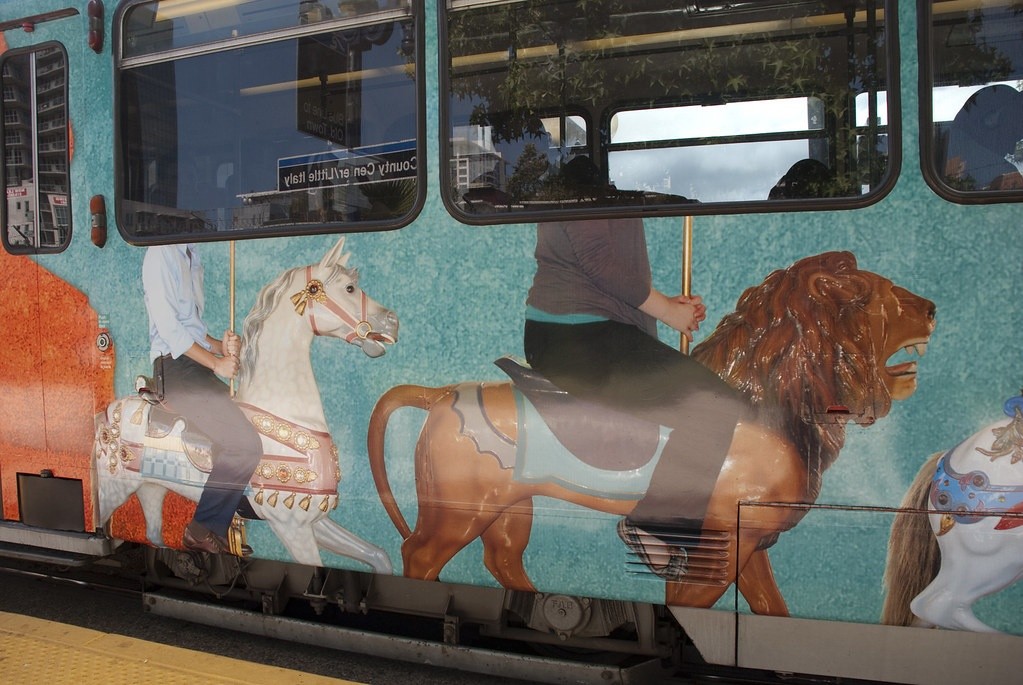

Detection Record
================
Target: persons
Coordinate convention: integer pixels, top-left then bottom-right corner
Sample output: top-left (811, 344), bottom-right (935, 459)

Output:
top-left (943, 84), bottom-right (1023, 190)
top-left (472, 109), bottom-right (578, 203)
top-left (142, 243), bottom-right (265, 559)
top-left (521, 221), bottom-right (742, 582)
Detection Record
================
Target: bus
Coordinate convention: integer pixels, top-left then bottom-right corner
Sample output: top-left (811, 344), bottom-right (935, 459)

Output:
top-left (0, 0), bottom-right (1023, 684)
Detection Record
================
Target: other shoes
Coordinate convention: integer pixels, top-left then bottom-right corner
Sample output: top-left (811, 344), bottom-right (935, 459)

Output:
top-left (182, 524), bottom-right (230, 555)
top-left (618, 517), bottom-right (688, 580)
